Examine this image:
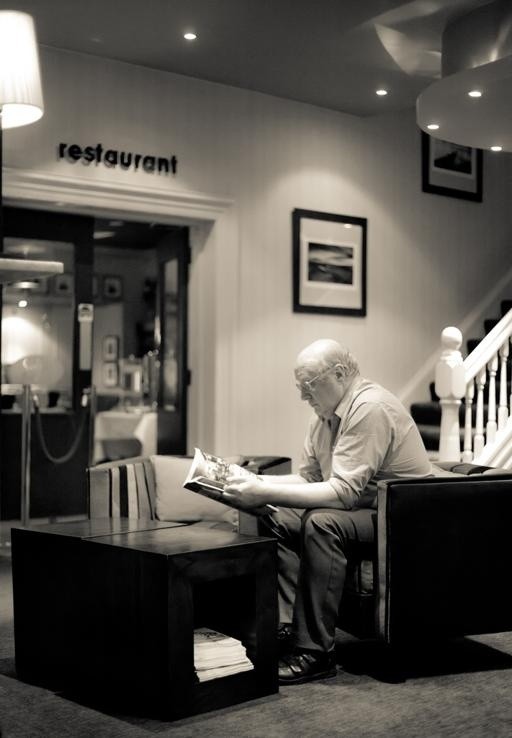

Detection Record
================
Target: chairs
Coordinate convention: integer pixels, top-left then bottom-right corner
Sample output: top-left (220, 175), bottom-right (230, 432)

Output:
top-left (86, 454), bottom-right (290, 535)
top-left (335, 462), bottom-right (512, 683)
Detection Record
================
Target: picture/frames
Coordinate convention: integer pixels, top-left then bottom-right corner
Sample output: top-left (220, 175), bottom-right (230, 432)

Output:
top-left (421, 130), bottom-right (484, 203)
top-left (292, 208), bottom-right (367, 317)
top-left (101, 336), bottom-right (119, 389)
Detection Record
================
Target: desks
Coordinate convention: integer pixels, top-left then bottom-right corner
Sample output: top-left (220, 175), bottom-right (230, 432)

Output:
top-left (94, 411), bottom-right (158, 461)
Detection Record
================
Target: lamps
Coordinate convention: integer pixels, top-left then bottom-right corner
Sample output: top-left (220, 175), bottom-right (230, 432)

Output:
top-left (0, 10), bottom-right (44, 130)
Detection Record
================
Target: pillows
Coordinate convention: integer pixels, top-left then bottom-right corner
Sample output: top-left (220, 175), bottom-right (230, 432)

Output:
top-left (150, 454), bottom-right (242, 525)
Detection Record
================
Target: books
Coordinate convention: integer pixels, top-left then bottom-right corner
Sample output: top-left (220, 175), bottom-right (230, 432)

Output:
top-left (179, 446), bottom-right (280, 516)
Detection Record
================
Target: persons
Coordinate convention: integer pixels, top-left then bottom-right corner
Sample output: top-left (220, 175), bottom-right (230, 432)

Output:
top-left (213, 336), bottom-right (438, 687)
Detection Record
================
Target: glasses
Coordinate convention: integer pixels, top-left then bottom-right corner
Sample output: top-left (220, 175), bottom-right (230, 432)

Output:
top-left (295, 375), bottom-right (320, 393)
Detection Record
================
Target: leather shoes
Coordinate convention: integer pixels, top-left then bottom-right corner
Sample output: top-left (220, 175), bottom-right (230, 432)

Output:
top-left (278, 624), bottom-right (293, 643)
top-left (278, 652), bottom-right (337, 685)
